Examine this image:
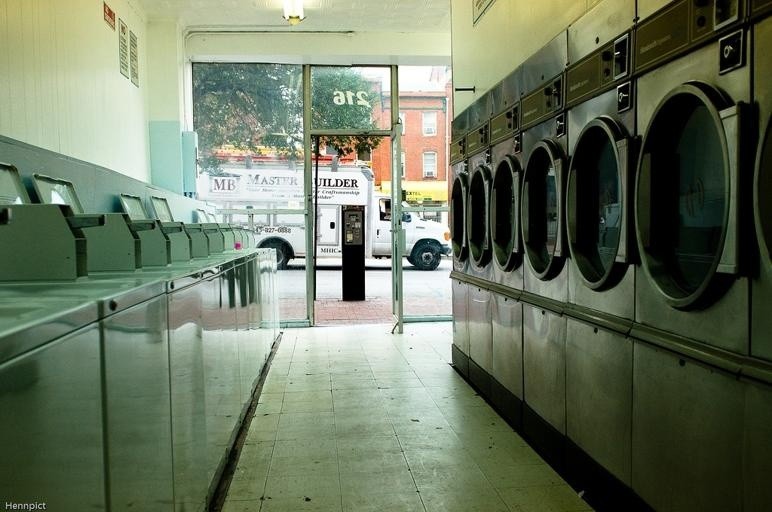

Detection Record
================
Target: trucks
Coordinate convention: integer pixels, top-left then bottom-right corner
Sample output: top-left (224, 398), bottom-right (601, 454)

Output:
top-left (200, 249), bottom-right (276, 281)
top-left (598, 202), bottom-right (725, 281)
top-left (195, 154), bottom-right (451, 271)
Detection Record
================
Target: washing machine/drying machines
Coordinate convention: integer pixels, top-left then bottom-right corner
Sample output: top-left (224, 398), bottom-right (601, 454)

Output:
top-left (448, 0), bottom-right (772, 382)
top-left (0, 130), bottom-right (283, 511)
